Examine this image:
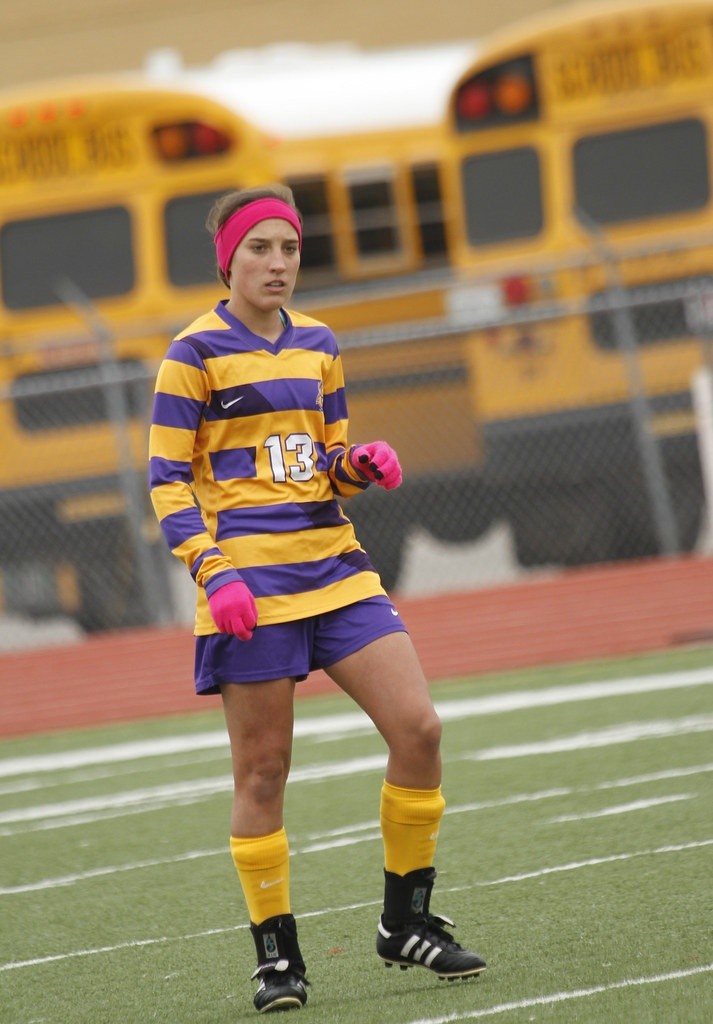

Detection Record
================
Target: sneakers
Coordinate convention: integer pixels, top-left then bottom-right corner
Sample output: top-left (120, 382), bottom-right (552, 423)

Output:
top-left (250, 959), bottom-right (311, 1013)
top-left (377, 913), bottom-right (487, 983)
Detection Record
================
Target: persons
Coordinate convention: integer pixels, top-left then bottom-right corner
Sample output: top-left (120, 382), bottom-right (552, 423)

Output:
top-left (147, 185), bottom-right (485, 1014)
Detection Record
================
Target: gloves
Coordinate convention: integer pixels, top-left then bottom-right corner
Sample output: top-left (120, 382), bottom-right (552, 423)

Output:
top-left (351, 441), bottom-right (403, 491)
top-left (208, 582), bottom-right (258, 641)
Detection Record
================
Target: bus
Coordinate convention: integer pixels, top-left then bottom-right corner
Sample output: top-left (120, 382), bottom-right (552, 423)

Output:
top-left (0, 0), bottom-right (713, 638)
